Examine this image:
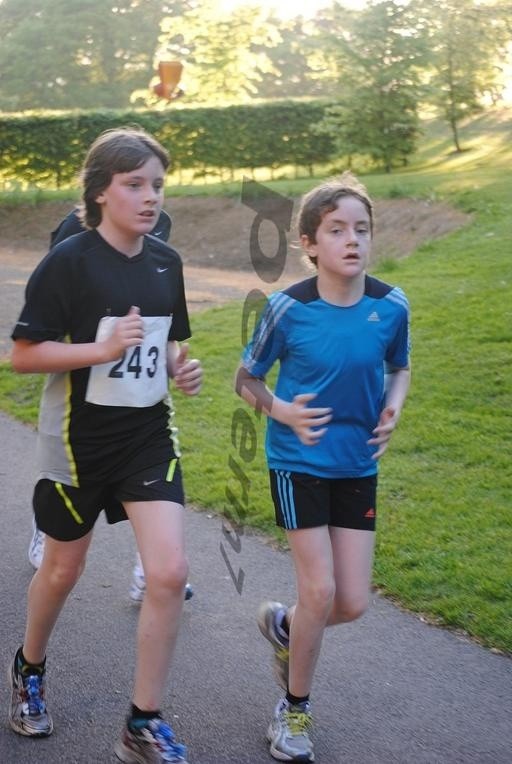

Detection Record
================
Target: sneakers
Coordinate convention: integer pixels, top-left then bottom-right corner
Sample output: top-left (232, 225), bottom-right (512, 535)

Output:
top-left (113, 716), bottom-right (191, 764)
top-left (257, 601), bottom-right (289, 692)
top-left (128, 551), bottom-right (194, 604)
top-left (8, 647), bottom-right (54, 738)
top-left (28, 513), bottom-right (48, 568)
top-left (266, 696), bottom-right (316, 763)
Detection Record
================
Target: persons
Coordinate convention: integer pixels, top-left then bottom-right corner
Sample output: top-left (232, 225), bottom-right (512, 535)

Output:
top-left (5, 122), bottom-right (204, 764)
top-left (236, 171), bottom-right (414, 764)
top-left (25, 203), bottom-right (198, 603)
